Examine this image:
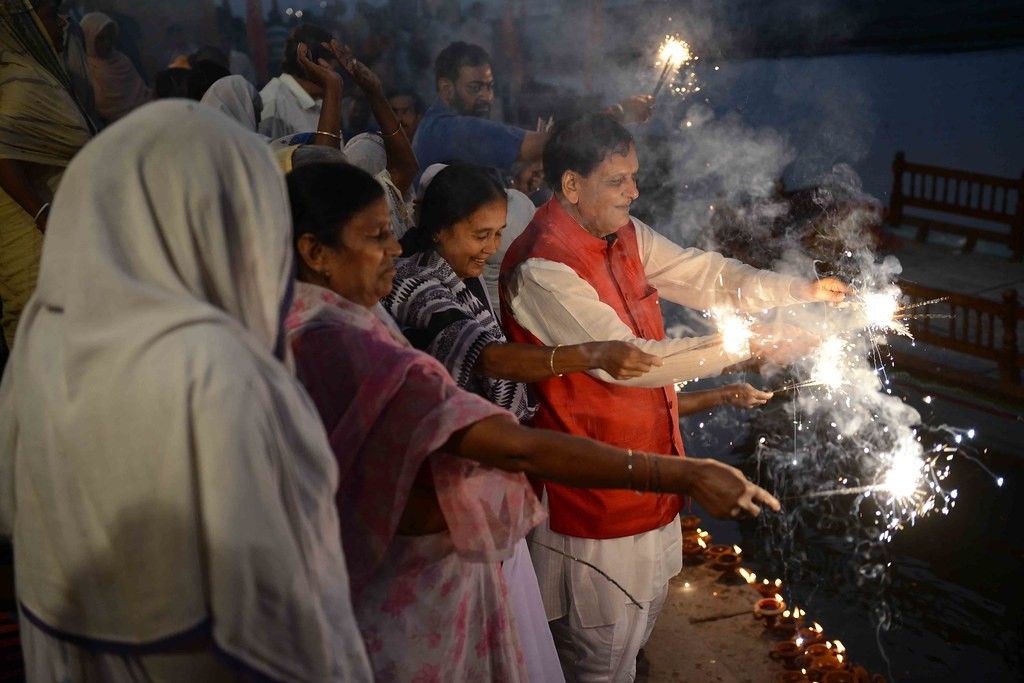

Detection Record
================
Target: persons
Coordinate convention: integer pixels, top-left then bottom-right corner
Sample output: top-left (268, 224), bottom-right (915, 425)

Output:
top-left (377, 162), bottom-right (664, 428)
top-left (0, 0), bottom-right (104, 356)
top-left (284, 160), bottom-right (783, 683)
top-left (0, 99), bottom-right (376, 683)
top-left (498, 115), bottom-right (860, 683)
top-left (653, 292), bottom-right (779, 578)
top-left (410, 40), bottom-right (656, 199)
top-left (61, 11), bottom-right (422, 193)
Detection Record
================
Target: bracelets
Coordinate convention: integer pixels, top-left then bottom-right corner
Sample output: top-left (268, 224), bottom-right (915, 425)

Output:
top-left (383, 122), bottom-right (401, 139)
top-left (316, 131), bottom-right (342, 141)
top-left (550, 343), bottom-right (564, 377)
top-left (33, 203), bottom-right (51, 228)
top-left (625, 448), bottom-right (666, 496)
